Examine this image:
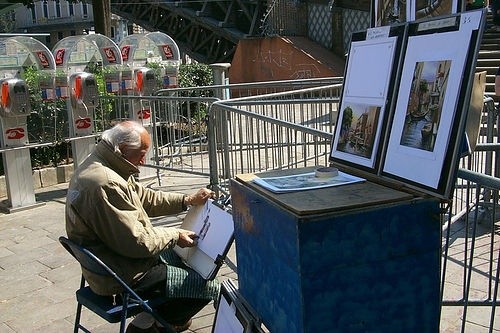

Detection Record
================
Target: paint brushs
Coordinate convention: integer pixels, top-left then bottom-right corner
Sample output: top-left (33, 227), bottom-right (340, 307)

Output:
top-left (189, 235), bottom-right (200, 239)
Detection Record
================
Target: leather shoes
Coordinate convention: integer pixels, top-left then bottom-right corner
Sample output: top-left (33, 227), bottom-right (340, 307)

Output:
top-left (126, 318), bottom-right (193, 333)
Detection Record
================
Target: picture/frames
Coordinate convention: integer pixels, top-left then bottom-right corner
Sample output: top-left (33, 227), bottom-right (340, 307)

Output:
top-left (329, 7), bottom-right (488, 202)
top-left (212, 278), bottom-right (266, 333)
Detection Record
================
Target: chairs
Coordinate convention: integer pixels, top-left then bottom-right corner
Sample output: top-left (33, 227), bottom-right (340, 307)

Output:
top-left (58, 236), bottom-right (177, 333)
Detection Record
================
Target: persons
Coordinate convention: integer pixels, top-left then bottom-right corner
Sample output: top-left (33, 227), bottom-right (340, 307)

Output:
top-left (65, 120), bottom-right (220, 333)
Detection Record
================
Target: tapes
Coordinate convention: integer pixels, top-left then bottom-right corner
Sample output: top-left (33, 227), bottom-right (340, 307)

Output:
top-left (314, 167), bottom-right (338, 178)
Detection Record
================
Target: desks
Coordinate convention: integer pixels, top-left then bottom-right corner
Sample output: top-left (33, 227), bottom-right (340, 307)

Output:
top-left (228, 165), bottom-right (441, 333)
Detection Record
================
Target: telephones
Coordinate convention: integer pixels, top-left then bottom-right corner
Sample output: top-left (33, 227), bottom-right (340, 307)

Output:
top-left (0, 77), bottom-right (31, 118)
top-left (132, 68), bottom-right (156, 96)
top-left (68, 72), bottom-right (99, 110)
